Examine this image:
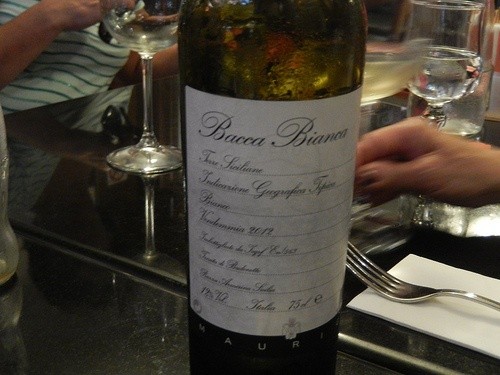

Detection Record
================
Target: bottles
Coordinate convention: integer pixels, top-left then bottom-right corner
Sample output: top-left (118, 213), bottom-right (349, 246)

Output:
top-left (176, 1), bottom-right (369, 375)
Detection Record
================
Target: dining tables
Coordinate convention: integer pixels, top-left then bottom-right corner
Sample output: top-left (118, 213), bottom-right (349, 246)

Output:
top-left (0, 42), bottom-right (500, 375)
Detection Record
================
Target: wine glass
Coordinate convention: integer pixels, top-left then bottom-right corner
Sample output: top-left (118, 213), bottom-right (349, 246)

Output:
top-left (399, 1), bottom-right (486, 137)
top-left (116, 170), bottom-right (186, 338)
top-left (99, 0), bottom-right (184, 173)
top-left (400, 192), bottom-right (469, 236)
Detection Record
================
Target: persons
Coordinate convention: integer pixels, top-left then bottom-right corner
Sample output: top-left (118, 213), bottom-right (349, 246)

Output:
top-left (0, 0), bottom-right (179, 116)
top-left (354, 114), bottom-right (500, 202)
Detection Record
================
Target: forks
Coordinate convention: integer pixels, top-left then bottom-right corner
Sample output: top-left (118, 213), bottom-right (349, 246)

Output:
top-left (345, 242), bottom-right (500, 311)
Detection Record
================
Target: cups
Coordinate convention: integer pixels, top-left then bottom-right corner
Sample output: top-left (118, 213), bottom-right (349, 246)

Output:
top-left (0, 285), bottom-right (26, 374)
top-left (0, 106), bottom-right (19, 284)
